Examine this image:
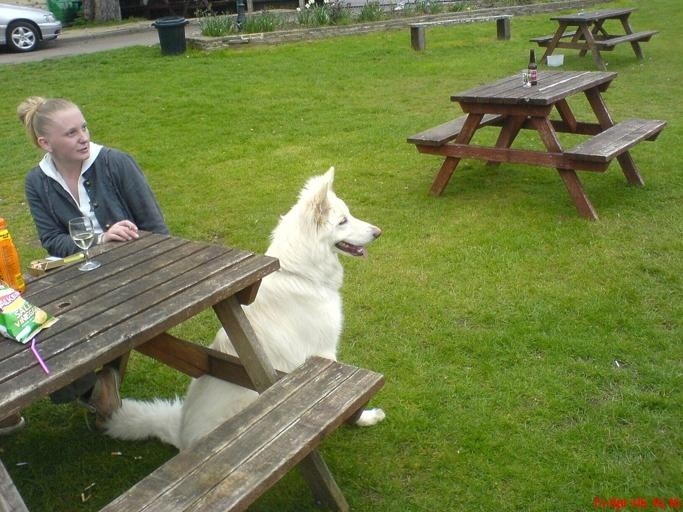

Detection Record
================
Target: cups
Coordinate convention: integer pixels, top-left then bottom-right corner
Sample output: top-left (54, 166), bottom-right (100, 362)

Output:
top-left (521, 68), bottom-right (530, 88)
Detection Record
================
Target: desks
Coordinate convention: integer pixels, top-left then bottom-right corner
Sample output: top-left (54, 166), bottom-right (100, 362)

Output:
top-left (1, 229), bottom-right (385, 512)
top-left (428, 71), bottom-right (645, 221)
top-left (539, 7), bottom-right (645, 72)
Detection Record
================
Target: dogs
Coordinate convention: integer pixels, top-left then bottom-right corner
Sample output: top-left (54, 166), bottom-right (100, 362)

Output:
top-left (94, 164), bottom-right (389, 458)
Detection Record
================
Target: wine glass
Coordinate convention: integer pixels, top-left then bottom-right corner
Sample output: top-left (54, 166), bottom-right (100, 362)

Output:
top-left (67, 215), bottom-right (101, 272)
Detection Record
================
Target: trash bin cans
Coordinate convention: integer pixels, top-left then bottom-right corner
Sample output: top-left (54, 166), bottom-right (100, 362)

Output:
top-left (151, 16), bottom-right (190, 57)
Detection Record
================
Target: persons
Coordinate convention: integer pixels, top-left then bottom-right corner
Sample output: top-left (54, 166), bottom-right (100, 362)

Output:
top-left (14, 95), bottom-right (171, 431)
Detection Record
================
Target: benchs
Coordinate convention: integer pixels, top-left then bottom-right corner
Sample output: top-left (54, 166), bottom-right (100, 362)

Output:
top-left (563, 120), bottom-right (667, 173)
top-left (95, 356), bottom-right (385, 512)
top-left (408, 15), bottom-right (513, 51)
top-left (594, 29), bottom-right (660, 50)
top-left (406, 113), bottom-right (503, 154)
top-left (529, 30), bottom-right (576, 46)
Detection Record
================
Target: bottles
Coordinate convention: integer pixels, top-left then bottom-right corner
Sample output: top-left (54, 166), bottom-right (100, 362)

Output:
top-left (0, 218), bottom-right (28, 296)
top-left (527, 48), bottom-right (538, 86)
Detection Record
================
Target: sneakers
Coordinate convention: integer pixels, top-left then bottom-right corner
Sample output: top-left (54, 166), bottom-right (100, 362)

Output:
top-left (89, 366), bottom-right (121, 431)
top-left (0, 411), bottom-right (25, 437)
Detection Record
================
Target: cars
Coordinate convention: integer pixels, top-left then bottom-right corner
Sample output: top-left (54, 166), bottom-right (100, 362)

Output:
top-left (0, 4), bottom-right (62, 51)
